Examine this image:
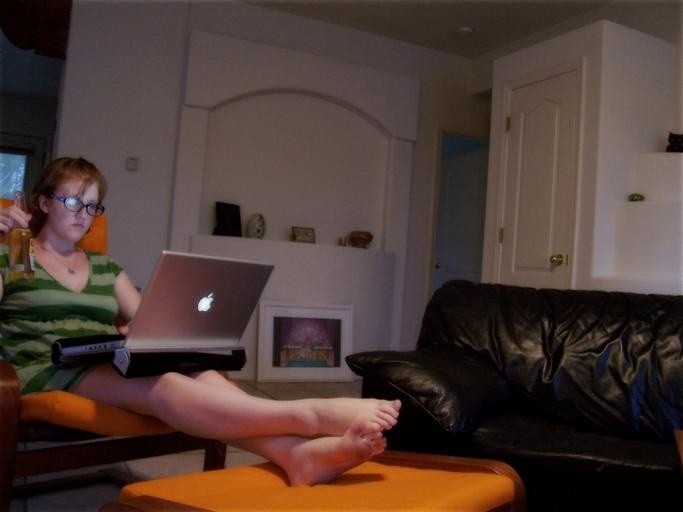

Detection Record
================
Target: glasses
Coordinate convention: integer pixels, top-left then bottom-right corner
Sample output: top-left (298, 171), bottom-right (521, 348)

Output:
top-left (52, 195), bottom-right (104, 217)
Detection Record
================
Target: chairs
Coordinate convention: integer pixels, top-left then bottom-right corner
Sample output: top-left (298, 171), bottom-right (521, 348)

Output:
top-left (0, 196), bottom-right (235, 510)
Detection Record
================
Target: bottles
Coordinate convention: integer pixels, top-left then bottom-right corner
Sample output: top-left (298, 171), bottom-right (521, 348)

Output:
top-left (8, 191), bottom-right (36, 284)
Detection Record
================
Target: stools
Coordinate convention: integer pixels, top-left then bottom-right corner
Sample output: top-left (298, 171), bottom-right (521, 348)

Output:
top-left (116, 450), bottom-right (528, 511)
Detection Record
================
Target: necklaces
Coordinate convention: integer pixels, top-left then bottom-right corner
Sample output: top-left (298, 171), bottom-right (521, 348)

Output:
top-left (38, 237), bottom-right (81, 275)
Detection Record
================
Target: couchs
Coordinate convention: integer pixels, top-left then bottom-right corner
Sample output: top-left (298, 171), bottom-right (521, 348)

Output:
top-left (343, 277), bottom-right (683, 511)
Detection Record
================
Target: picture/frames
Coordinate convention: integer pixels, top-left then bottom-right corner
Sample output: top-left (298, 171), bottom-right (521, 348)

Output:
top-left (256, 302), bottom-right (352, 381)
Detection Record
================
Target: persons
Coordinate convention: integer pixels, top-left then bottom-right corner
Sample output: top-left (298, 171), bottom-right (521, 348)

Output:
top-left (0, 155), bottom-right (401, 488)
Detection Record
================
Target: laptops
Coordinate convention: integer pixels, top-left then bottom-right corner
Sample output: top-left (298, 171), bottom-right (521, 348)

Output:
top-left (51, 250), bottom-right (274, 378)
